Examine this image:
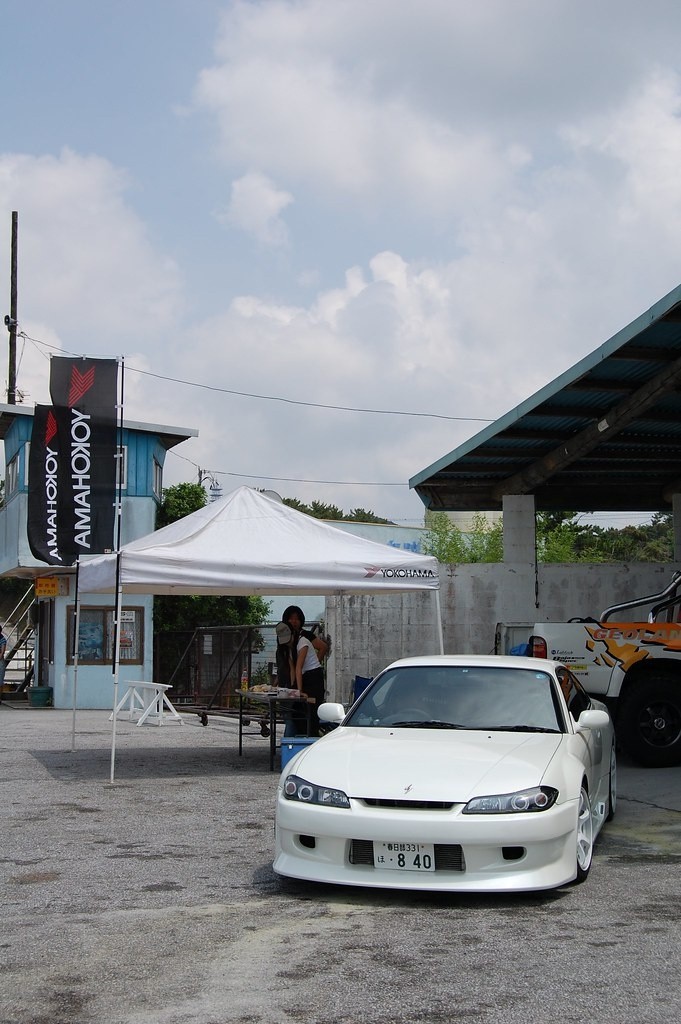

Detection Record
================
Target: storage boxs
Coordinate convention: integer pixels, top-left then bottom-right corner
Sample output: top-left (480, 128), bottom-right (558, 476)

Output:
top-left (280, 736), bottom-right (320, 773)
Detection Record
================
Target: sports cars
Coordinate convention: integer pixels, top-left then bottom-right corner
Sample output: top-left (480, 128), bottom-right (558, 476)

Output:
top-left (271, 656), bottom-right (615, 894)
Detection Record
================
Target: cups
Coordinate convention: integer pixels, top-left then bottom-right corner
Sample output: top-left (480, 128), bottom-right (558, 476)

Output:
top-left (277, 687), bottom-right (288, 698)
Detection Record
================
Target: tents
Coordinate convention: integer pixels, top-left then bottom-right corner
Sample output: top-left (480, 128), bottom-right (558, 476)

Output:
top-left (70, 486), bottom-right (446, 783)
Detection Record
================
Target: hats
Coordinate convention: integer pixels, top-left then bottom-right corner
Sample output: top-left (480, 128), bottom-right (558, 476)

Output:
top-left (275, 621), bottom-right (293, 645)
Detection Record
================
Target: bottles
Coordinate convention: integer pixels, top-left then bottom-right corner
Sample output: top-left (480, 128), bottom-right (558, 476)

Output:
top-left (241, 669), bottom-right (248, 691)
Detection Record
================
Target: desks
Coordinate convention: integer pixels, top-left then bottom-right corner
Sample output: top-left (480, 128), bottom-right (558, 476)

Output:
top-left (235, 689), bottom-right (314, 771)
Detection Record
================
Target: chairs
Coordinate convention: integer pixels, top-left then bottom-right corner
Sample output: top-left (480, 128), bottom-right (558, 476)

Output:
top-left (345, 675), bottom-right (374, 714)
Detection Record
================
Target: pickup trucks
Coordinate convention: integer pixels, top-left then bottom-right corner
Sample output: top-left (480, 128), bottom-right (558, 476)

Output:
top-left (493, 573), bottom-right (681, 769)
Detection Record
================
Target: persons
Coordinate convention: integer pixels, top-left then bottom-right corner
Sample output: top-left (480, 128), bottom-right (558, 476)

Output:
top-left (275, 606), bottom-right (328, 737)
top-left (0, 626), bottom-right (7, 705)
